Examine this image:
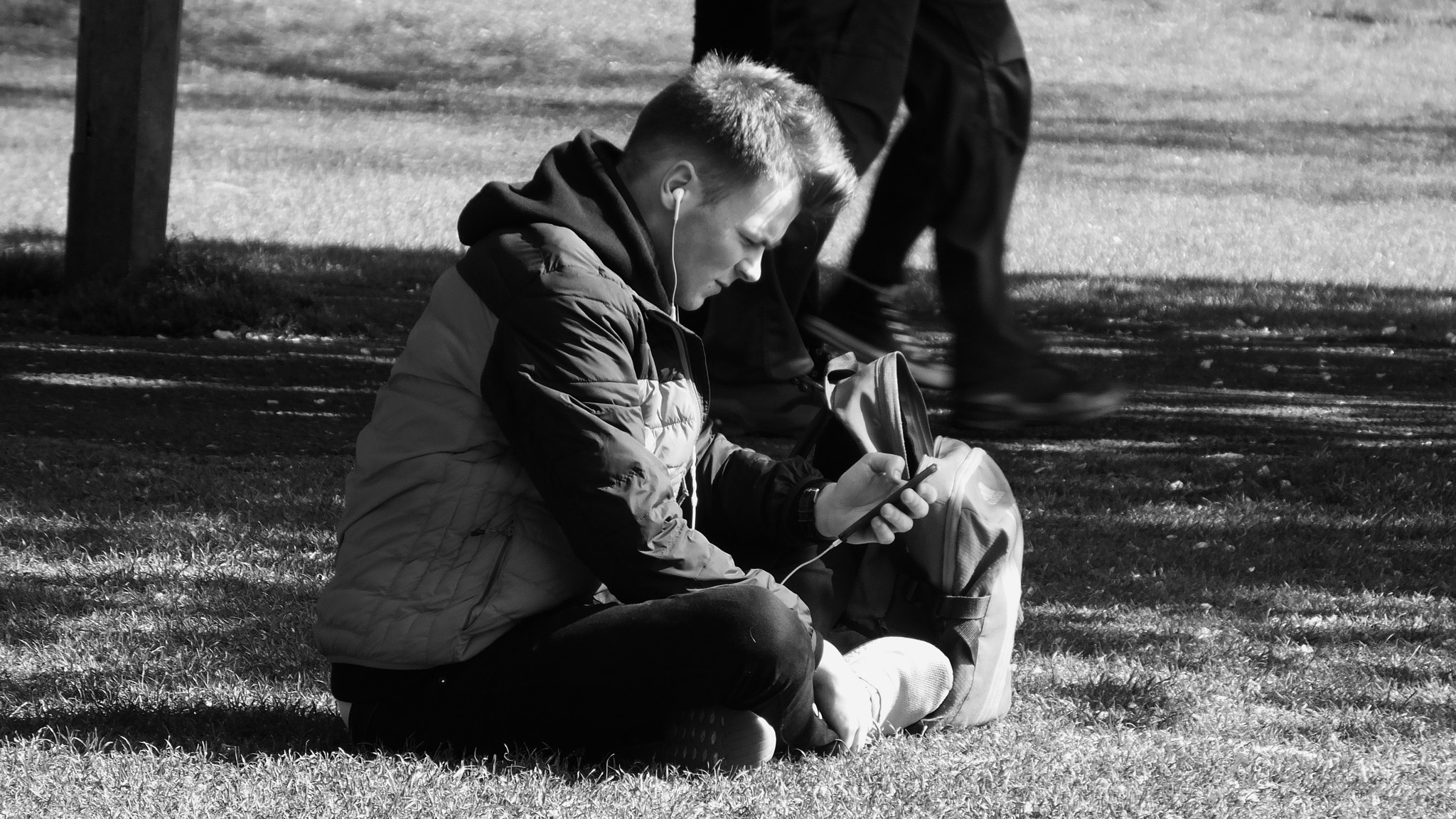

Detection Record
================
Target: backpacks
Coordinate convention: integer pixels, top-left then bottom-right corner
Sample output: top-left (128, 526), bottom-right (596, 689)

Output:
top-left (823, 351), bottom-right (1023, 732)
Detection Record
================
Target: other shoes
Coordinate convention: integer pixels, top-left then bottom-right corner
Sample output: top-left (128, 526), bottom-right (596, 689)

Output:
top-left (819, 634), bottom-right (952, 734)
top-left (634, 701), bottom-right (779, 772)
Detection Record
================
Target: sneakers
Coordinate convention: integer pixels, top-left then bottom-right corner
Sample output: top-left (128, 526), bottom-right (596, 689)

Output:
top-left (963, 353), bottom-right (1121, 435)
top-left (805, 271), bottom-right (954, 390)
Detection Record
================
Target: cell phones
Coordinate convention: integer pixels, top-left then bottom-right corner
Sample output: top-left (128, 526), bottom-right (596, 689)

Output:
top-left (838, 463), bottom-right (937, 543)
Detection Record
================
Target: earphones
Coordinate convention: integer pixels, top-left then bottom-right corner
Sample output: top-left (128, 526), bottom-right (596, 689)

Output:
top-left (673, 187), bottom-right (685, 222)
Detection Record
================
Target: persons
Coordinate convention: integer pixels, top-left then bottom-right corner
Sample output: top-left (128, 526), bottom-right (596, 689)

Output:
top-left (820, 0), bottom-right (1131, 433)
top-left (312, 45), bottom-right (955, 774)
top-left (686, 0), bottom-right (920, 437)
